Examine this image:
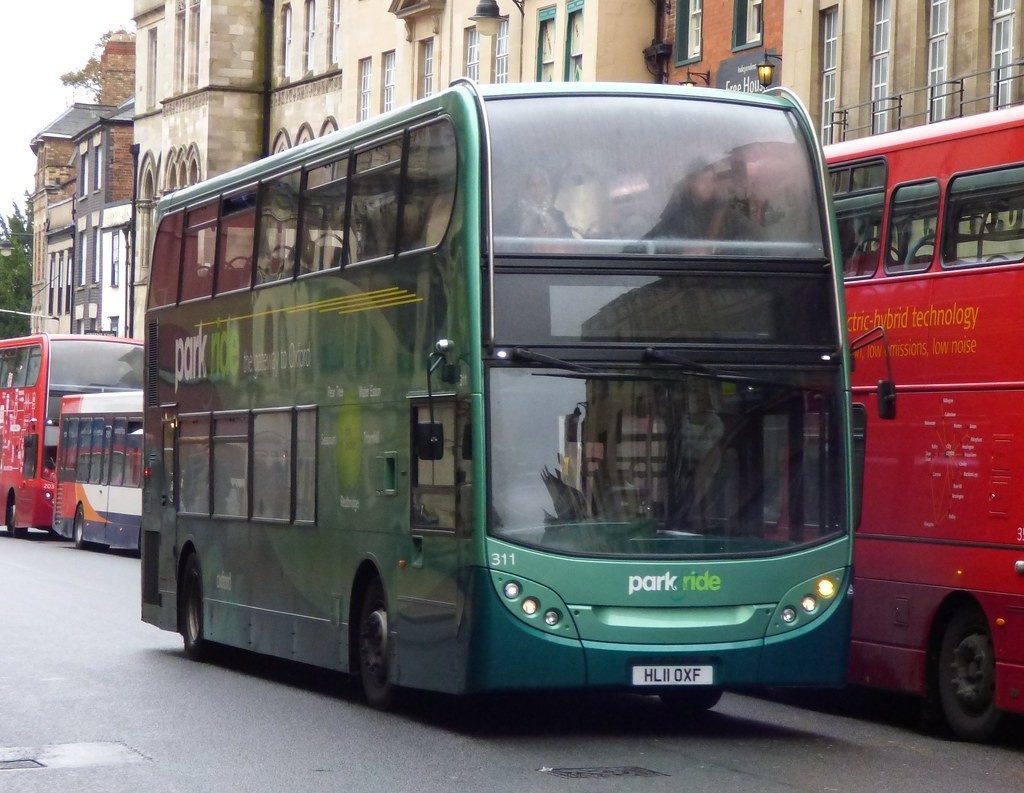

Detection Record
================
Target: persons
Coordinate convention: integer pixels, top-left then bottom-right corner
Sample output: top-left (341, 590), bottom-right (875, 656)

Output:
top-left (664, 157), bottom-right (767, 242)
top-left (494, 171), bottom-right (576, 238)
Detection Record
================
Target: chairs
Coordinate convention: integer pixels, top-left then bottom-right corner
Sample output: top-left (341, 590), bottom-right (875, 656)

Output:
top-left (850, 231), bottom-right (1009, 275)
top-left (192, 230), bottom-right (354, 298)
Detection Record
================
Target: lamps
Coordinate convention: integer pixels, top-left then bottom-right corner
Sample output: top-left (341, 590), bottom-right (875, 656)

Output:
top-left (468, 0), bottom-right (525, 37)
top-left (755, 48), bottom-right (783, 90)
top-left (679, 67), bottom-right (711, 87)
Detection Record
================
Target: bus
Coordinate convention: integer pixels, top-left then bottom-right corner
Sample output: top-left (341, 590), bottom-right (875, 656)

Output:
top-left (564, 102), bottom-right (1023, 746)
top-left (52, 391), bottom-right (315, 548)
top-left (142, 77), bottom-right (898, 717)
top-left (0, 333), bottom-right (144, 538)
